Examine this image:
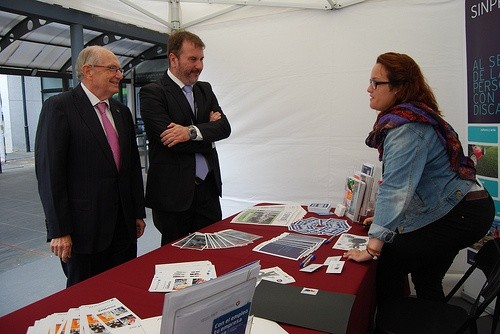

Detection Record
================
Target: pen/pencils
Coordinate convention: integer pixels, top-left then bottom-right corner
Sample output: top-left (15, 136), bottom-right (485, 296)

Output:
top-left (325, 234), bottom-right (336, 244)
top-left (358, 223), bottom-right (370, 226)
top-left (303, 255), bottom-right (314, 267)
top-left (300, 254), bottom-right (312, 266)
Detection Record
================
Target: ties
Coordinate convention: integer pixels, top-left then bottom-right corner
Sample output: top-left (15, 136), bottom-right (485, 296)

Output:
top-left (95, 103), bottom-right (121, 173)
top-left (183, 86), bottom-right (209, 181)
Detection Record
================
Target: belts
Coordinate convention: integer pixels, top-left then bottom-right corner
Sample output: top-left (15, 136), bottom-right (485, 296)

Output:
top-left (464, 191), bottom-right (488, 201)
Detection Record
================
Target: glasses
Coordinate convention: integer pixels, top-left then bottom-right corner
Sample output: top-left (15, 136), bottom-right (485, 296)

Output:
top-left (369, 78), bottom-right (391, 90)
top-left (87, 64), bottom-right (123, 73)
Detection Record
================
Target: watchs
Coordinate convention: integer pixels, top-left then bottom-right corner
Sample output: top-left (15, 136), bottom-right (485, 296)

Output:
top-left (188, 125), bottom-right (197, 139)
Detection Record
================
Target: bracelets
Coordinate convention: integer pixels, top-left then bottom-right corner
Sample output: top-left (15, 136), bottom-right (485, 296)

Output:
top-left (366, 245), bottom-right (381, 260)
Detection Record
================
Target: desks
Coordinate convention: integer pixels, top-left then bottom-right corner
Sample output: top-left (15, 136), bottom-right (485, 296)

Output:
top-left (0, 203), bottom-right (411, 334)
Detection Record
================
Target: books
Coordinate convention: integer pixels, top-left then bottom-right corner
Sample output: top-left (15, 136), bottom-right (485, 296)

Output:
top-left (344, 164), bottom-right (382, 222)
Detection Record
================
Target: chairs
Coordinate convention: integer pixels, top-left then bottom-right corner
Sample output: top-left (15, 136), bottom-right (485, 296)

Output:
top-left (375, 238), bottom-right (500, 334)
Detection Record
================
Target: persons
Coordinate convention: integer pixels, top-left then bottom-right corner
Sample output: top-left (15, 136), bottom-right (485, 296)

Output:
top-left (139, 31), bottom-right (231, 247)
top-left (34, 45), bottom-right (147, 290)
top-left (342, 52), bottom-right (496, 334)
